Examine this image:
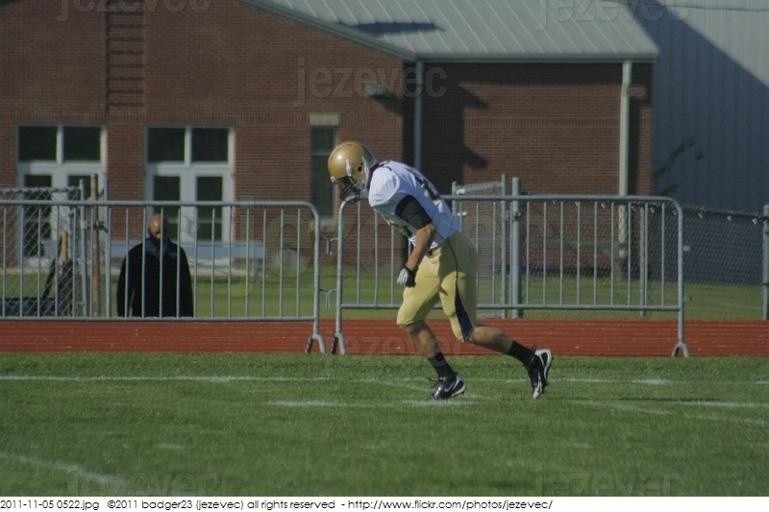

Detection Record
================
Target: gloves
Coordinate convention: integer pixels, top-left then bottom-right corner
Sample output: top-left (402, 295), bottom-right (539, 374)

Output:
top-left (395, 267), bottom-right (413, 286)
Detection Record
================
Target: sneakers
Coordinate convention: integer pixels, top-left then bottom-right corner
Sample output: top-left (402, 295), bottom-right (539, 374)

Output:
top-left (426, 375), bottom-right (466, 401)
top-left (527, 346), bottom-right (550, 398)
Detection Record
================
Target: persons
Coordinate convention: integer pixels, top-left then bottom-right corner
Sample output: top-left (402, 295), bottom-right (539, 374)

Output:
top-left (327, 140), bottom-right (555, 405)
top-left (117, 213), bottom-right (194, 317)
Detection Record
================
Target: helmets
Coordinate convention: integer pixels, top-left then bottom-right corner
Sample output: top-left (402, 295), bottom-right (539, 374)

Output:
top-left (327, 140), bottom-right (378, 206)
